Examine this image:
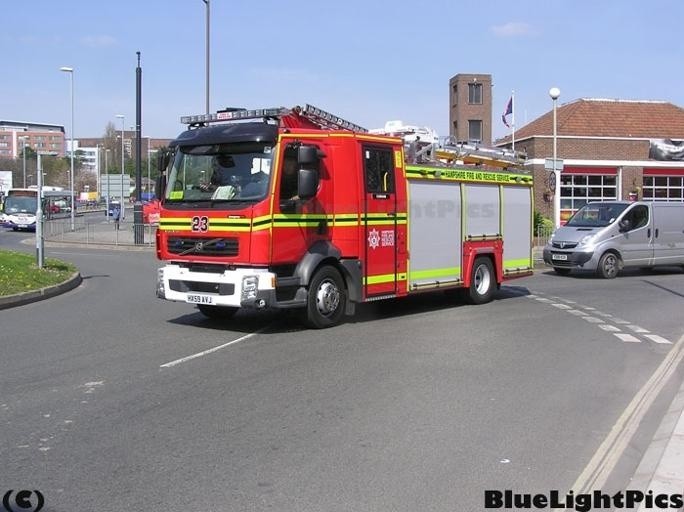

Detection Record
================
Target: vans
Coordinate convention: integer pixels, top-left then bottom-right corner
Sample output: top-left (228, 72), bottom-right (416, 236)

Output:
top-left (543, 196), bottom-right (684, 277)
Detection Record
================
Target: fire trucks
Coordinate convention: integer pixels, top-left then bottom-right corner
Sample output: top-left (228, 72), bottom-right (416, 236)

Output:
top-left (147, 101), bottom-right (539, 329)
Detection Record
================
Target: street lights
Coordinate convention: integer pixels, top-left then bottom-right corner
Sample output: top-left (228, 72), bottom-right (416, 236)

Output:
top-left (59, 66), bottom-right (78, 233)
top-left (114, 113), bottom-right (128, 221)
top-left (549, 86), bottom-right (563, 229)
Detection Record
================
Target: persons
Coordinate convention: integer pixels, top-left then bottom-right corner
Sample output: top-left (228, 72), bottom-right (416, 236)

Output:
top-left (112, 205), bottom-right (121, 229)
top-left (279, 157), bottom-right (300, 200)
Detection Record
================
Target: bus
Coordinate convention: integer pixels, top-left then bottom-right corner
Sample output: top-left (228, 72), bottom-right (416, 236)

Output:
top-left (4, 187), bottom-right (40, 232)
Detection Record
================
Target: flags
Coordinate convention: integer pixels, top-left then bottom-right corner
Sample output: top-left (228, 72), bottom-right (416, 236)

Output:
top-left (501, 96), bottom-right (512, 128)
